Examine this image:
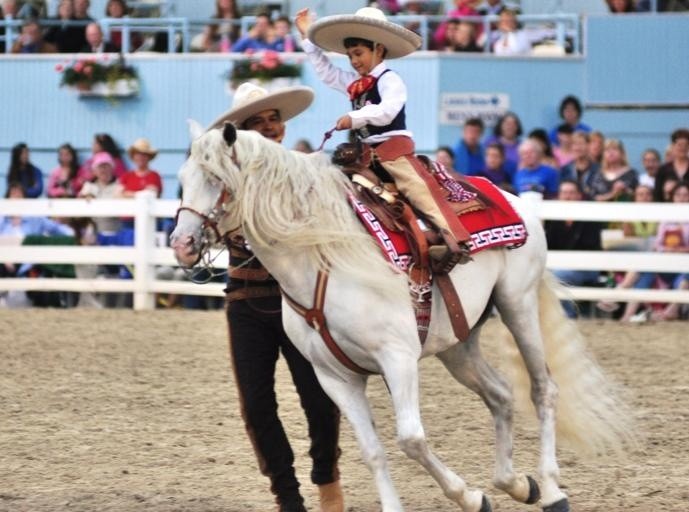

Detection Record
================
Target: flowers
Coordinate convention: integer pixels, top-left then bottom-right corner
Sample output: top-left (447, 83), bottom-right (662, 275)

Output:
top-left (52, 53), bottom-right (141, 105)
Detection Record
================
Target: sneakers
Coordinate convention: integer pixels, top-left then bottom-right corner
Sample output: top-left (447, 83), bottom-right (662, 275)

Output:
top-left (630, 309), bottom-right (651, 323)
top-left (429, 244), bottom-right (471, 259)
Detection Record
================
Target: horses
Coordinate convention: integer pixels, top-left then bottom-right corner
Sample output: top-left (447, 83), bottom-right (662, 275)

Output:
top-left (168, 117), bottom-right (650, 512)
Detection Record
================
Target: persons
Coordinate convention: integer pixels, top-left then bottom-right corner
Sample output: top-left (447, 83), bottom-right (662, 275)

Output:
top-left (2, 135), bottom-right (164, 311)
top-left (0, 0), bottom-right (689, 53)
top-left (434, 95), bottom-right (689, 324)
top-left (295, 6), bottom-right (472, 264)
top-left (202, 82), bottom-right (345, 512)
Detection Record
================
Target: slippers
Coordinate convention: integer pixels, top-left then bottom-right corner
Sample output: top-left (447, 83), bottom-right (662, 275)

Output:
top-left (597, 301), bottom-right (619, 313)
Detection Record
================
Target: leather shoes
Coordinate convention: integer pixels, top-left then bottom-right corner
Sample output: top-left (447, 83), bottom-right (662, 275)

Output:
top-left (318, 475), bottom-right (345, 512)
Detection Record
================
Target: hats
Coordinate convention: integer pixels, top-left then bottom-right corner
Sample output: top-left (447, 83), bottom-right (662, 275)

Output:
top-left (307, 6), bottom-right (422, 60)
top-left (126, 139), bottom-right (157, 158)
top-left (91, 151), bottom-right (115, 168)
top-left (206, 82), bottom-right (314, 128)
